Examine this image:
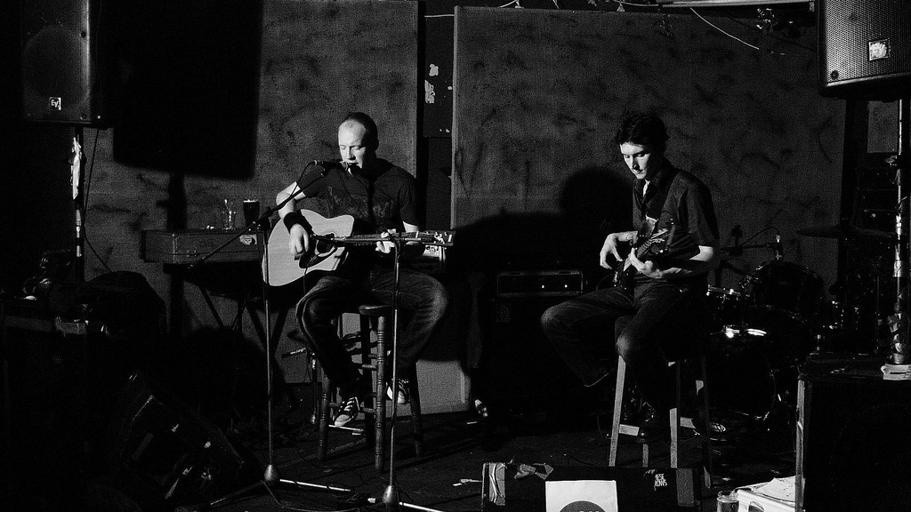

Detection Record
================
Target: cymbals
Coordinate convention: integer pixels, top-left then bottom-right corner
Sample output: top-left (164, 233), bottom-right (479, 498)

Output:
top-left (796, 217), bottom-right (891, 242)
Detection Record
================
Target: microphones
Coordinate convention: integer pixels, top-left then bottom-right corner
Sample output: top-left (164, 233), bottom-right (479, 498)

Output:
top-left (312, 158), bottom-right (352, 173)
top-left (772, 235), bottom-right (784, 262)
top-left (417, 229), bottom-right (459, 247)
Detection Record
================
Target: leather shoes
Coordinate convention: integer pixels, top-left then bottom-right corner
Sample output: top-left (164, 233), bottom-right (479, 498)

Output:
top-left (623, 384), bottom-right (657, 424)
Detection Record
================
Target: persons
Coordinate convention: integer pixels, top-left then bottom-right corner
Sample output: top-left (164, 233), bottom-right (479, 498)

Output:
top-left (275, 110), bottom-right (448, 428)
top-left (541, 110), bottom-right (720, 429)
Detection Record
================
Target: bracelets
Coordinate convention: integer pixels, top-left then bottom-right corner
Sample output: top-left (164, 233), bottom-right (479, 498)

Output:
top-left (283, 210), bottom-right (301, 234)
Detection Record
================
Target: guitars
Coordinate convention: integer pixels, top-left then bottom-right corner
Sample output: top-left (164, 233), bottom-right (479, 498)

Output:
top-left (600, 228), bottom-right (671, 289)
top-left (259, 209), bottom-right (458, 287)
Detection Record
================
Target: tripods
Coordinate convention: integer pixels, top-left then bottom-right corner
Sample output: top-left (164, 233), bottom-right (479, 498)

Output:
top-left (331, 249), bottom-right (445, 512)
top-left (171, 297), bottom-right (379, 512)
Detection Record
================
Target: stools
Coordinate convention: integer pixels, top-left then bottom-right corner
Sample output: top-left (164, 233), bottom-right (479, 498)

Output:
top-left (312, 296), bottom-right (427, 476)
top-left (607, 312), bottom-right (714, 492)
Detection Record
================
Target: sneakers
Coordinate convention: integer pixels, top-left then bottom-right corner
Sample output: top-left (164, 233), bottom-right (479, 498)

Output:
top-left (331, 395), bottom-right (360, 426)
top-left (385, 376), bottom-right (410, 404)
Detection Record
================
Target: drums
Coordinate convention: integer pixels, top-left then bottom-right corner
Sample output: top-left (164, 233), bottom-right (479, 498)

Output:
top-left (712, 321), bottom-right (800, 430)
top-left (753, 259), bottom-right (825, 352)
top-left (825, 301), bottom-right (880, 333)
top-left (706, 285), bottom-right (751, 329)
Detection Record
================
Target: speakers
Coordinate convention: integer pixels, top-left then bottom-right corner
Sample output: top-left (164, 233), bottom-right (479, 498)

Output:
top-left (820, 2), bottom-right (909, 101)
top-left (793, 377), bottom-right (911, 511)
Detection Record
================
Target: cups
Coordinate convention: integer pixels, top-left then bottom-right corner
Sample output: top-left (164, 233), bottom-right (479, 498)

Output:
top-left (221, 199), bottom-right (236, 231)
top-left (244, 200), bottom-right (260, 229)
top-left (717, 490), bottom-right (740, 512)
